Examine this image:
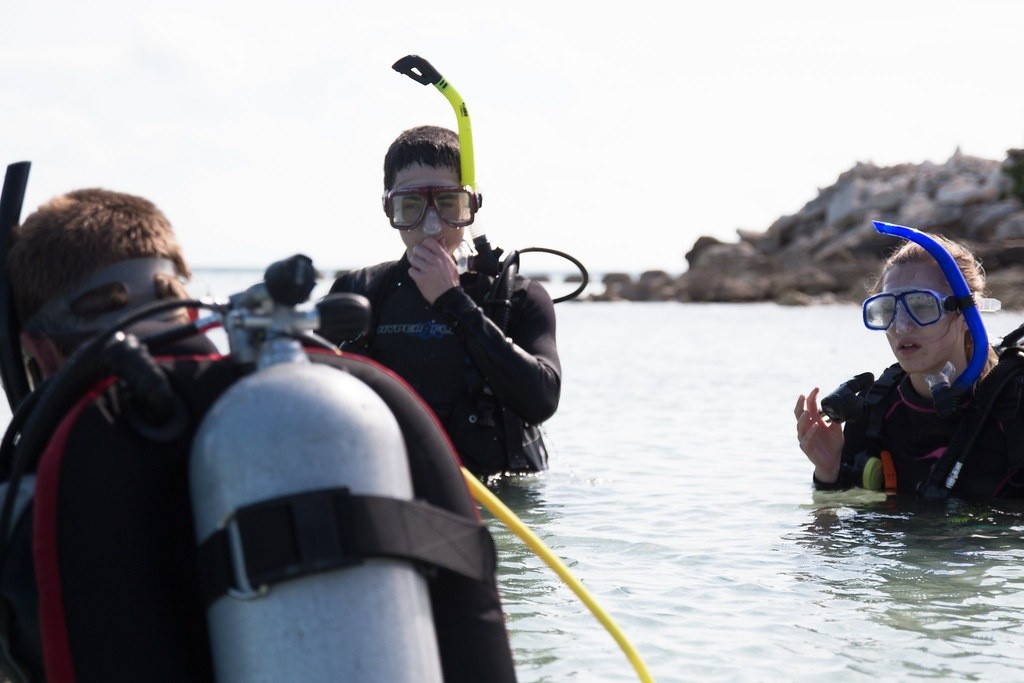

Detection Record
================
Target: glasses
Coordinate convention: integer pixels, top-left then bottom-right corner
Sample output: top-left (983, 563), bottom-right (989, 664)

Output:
top-left (862, 288), bottom-right (950, 329)
top-left (383, 186), bottom-right (479, 231)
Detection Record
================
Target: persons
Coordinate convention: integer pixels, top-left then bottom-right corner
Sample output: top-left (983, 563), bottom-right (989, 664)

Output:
top-left (0, 187), bottom-right (517, 683)
top-left (324, 125), bottom-right (561, 475)
top-left (794, 233), bottom-right (1024, 501)
